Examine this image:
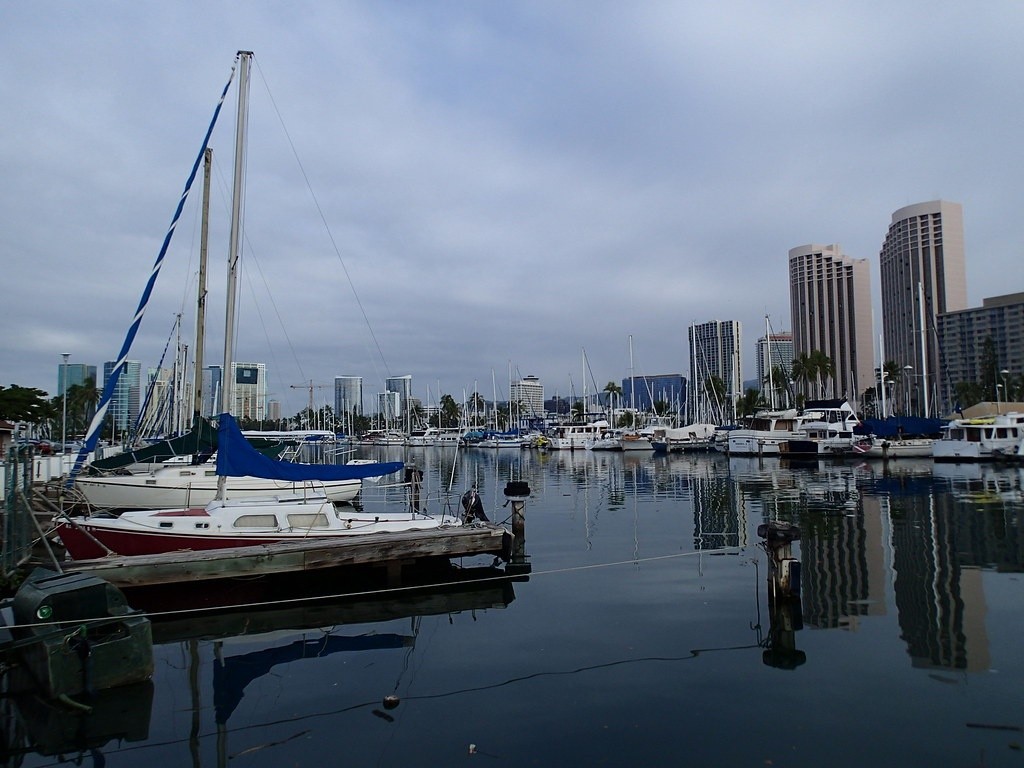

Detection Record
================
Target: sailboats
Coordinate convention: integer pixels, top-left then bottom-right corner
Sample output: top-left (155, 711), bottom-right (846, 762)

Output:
top-left (339, 282), bottom-right (1024, 468)
top-left (45, 50), bottom-right (463, 562)
